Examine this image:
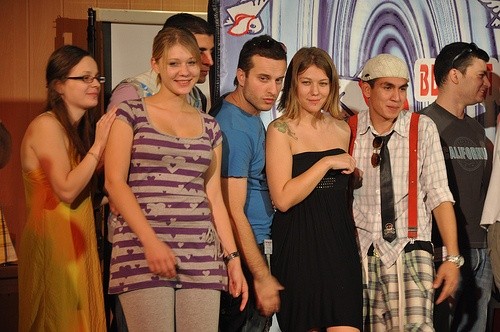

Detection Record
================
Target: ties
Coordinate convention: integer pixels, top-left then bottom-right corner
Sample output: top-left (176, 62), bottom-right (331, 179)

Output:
top-left (370, 130), bottom-right (399, 243)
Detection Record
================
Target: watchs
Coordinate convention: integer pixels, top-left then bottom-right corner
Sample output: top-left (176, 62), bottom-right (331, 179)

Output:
top-left (442, 254), bottom-right (465, 268)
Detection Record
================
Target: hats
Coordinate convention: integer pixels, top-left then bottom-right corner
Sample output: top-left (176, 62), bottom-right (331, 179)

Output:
top-left (357, 54), bottom-right (409, 81)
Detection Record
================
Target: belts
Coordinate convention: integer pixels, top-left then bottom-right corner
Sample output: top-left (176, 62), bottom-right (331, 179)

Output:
top-left (367, 240), bottom-right (434, 257)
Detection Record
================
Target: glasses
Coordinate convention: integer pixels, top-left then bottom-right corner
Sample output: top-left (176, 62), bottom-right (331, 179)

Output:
top-left (452, 41), bottom-right (478, 68)
top-left (371, 136), bottom-right (383, 168)
top-left (66, 74), bottom-right (106, 84)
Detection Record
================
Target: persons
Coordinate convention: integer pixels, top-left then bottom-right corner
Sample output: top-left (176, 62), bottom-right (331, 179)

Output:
top-left (208, 35), bottom-right (287, 332)
top-left (344, 53), bottom-right (465, 332)
top-left (106, 25), bottom-right (249, 332)
top-left (416, 42), bottom-right (496, 332)
top-left (107, 14), bottom-right (215, 115)
top-left (17, 45), bottom-right (117, 332)
top-left (265, 46), bottom-right (363, 332)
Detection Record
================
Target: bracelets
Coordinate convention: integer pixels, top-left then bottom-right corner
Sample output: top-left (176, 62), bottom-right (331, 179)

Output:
top-left (87, 151), bottom-right (100, 162)
top-left (222, 251), bottom-right (240, 265)
